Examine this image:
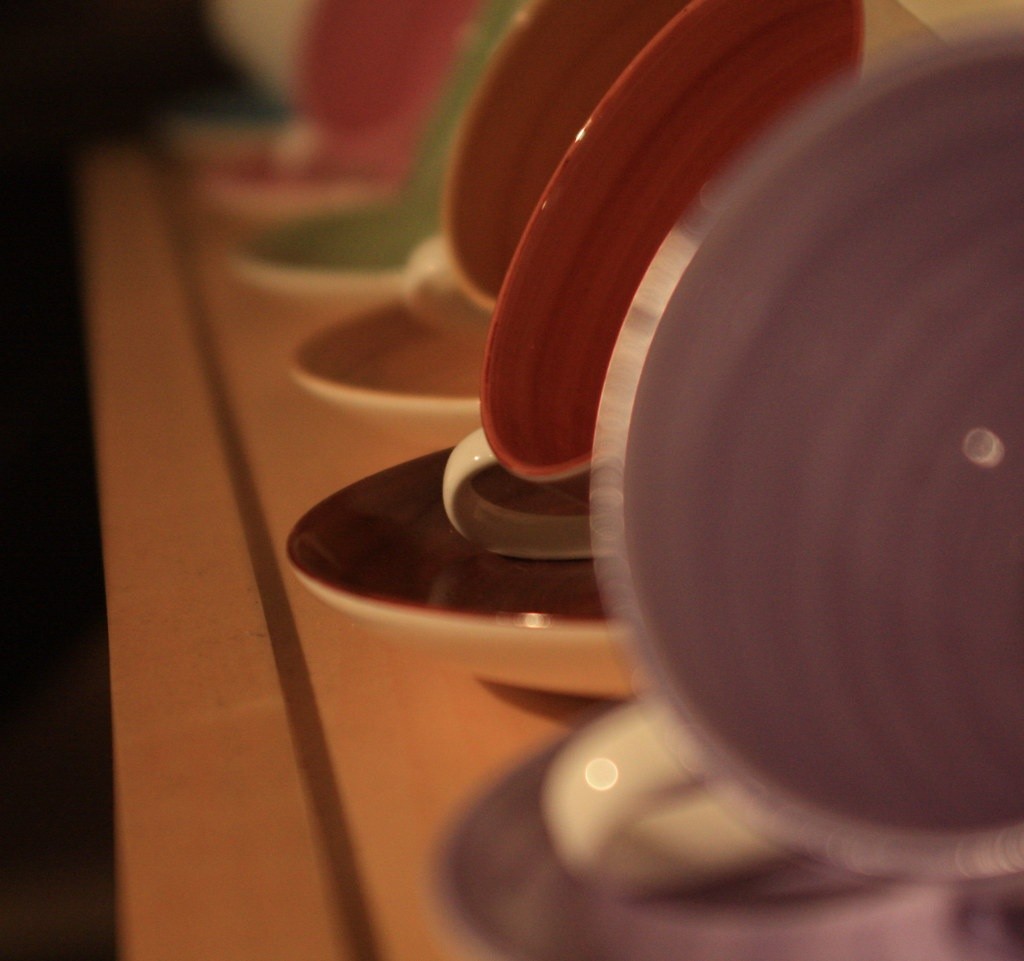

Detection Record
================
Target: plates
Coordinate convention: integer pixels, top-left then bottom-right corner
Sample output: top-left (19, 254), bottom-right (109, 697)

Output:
top-left (284, 446), bottom-right (644, 698)
top-left (429, 730), bottom-right (1020, 961)
top-left (293, 295), bottom-right (482, 430)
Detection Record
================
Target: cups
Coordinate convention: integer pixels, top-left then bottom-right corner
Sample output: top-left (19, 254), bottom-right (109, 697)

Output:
top-left (218, 0), bottom-right (1024, 902)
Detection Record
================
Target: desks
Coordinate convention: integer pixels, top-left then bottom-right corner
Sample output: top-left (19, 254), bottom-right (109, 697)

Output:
top-left (87, 117), bottom-right (629, 960)
